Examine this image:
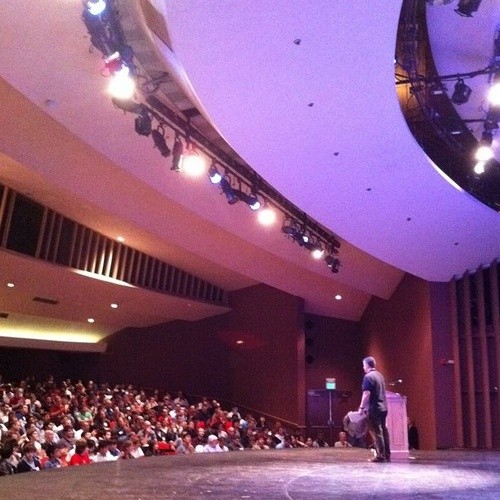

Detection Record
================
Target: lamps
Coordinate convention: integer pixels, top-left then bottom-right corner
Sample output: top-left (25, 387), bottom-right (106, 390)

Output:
top-left (453, 0), bottom-right (481, 18)
top-left (478, 130), bottom-right (494, 159)
top-left (81, 0), bottom-right (341, 275)
top-left (452, 83), bottom-right (471, 105)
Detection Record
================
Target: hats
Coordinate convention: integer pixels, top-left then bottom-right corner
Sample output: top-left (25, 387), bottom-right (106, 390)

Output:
top-left (108, 438), bottom-right (118, 444)
top-left (218, 431), bottom-right (228, 440)
top-left (208, 435), bottom-right (218, 442)
top-left (180, 430), bottom-right (192, 438)
top-left (118, 436), bottom-right (129, 444)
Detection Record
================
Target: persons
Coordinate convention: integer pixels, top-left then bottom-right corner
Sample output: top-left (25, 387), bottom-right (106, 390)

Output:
top-left (0, 375), bottom-right (308, 477)
top-left (407, 417), bottom-right (419, 449)
top-left (305, 436), bottom-right (314, 447)
top-left (334, 432), bottom-right (352, 448)
top-left (359, 356), bottom-right (391, 463)
top-left (312, 431), bottom-right (329, 448)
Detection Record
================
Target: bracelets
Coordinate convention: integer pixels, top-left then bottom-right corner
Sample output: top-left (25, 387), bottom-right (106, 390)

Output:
top-left (360, 407), bottom-right (364, 409)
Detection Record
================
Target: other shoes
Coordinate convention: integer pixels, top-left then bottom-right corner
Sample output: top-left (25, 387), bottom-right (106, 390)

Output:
top-left (368, 456), bottom-right (390, 462)
